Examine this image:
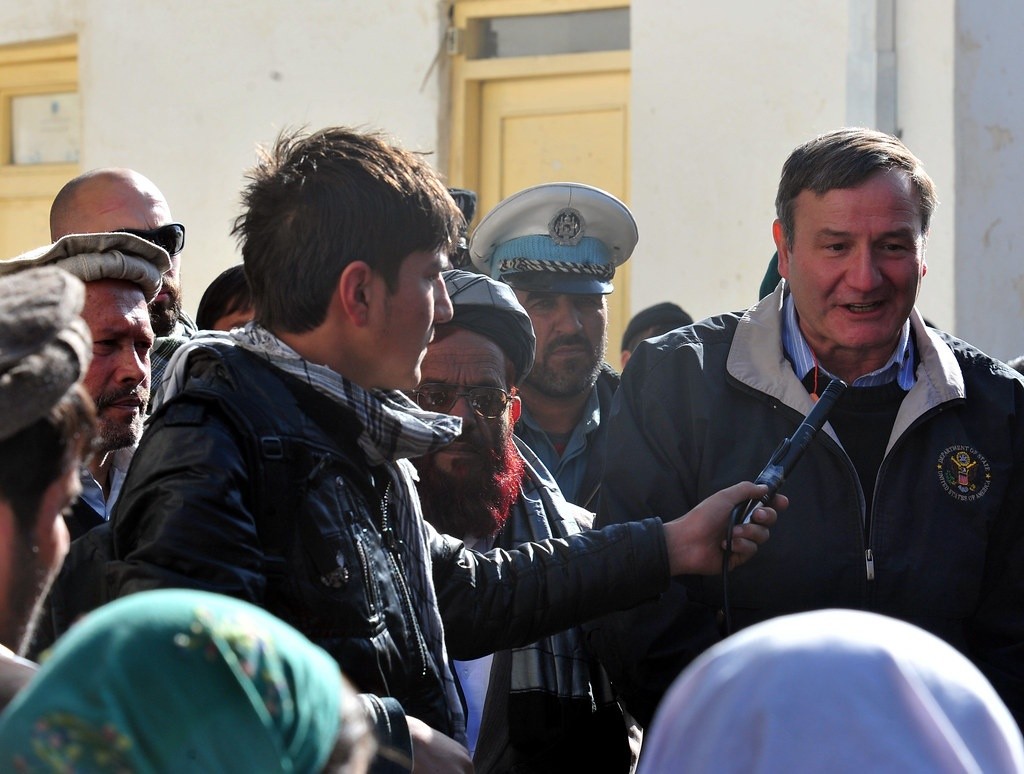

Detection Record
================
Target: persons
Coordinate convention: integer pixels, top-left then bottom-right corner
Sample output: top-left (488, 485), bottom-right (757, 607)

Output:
top-left (0, 585), bottom-right (380, 774)
top-left (106, 124), bottom-right (791, 774)
top-left (636, 606), bottom-right (1024, 774)
top-left (193, 262), bottom-right (259, 338)
top-left (47, 162), bottom-right (196, 413)
top-left (0, 226), bottom-right (173, 663)
top-left (439, 185), bottom-right (478, 274)
top-left (569, 128), bottom-right (1024, 733)
top-left (618, 298), bottom-right (695, 372)
top-left (466, 179), bottom-right (646, 523)
top-left (0, 261), bottom-right (103, 712)
top-left (390, 267), bottom-right (647, 773)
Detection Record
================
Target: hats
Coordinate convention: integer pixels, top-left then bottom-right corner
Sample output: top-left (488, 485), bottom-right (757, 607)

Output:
top-left (0, 264), bottom-right (92, 439)
top-left (425, 269), bottom-right (536, 392)
top-left (621, 302), bottom-right (694, 352)
top-left (0, 233), bottom-right (173, 303)
top-left (468, 183), bottom-right (638, 295)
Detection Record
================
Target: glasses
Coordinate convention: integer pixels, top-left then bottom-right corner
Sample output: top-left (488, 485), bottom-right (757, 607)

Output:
top-left (110, 223), bottom-right (185, 256)
top-left (415, 382), bottom-right (516, 419)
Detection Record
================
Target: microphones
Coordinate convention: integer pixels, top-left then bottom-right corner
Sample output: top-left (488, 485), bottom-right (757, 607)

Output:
top-left (739, 379), bottom-right (847, 526)
